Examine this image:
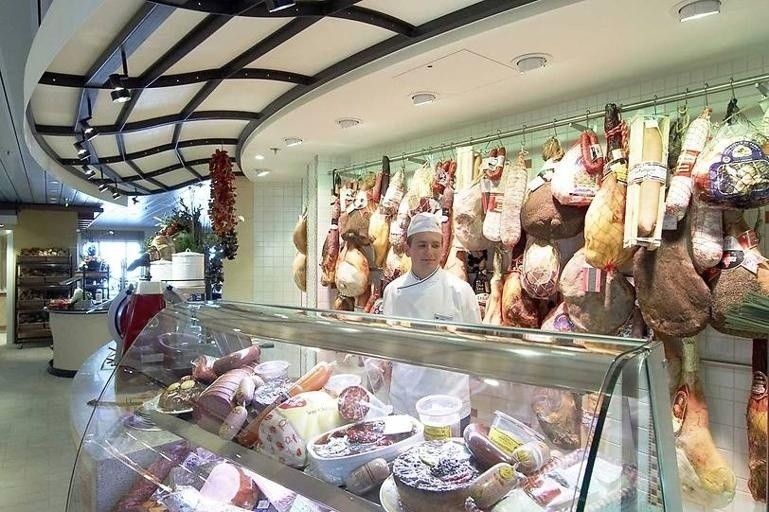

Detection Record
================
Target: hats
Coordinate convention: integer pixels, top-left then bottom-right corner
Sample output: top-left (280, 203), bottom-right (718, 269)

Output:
top-left (404, 212), bottom-right (444, 237)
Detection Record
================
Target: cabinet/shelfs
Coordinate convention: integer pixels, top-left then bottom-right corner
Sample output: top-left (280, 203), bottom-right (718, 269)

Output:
top-left (67, 300), bottom-right (683, 512)
top-left (12, 248), bottom-right (72, 349)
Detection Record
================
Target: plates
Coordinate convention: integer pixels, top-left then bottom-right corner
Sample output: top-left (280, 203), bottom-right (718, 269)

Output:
top-left (379, 473), bottom-right (404, 512)
top-left (154, 395), bottom-right (193, 414)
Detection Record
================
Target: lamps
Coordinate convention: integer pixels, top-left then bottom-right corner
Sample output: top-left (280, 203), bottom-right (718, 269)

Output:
top-left (109, 48), bottom-right (132, 102)
top-left (73, 131), bottom-right (92, 161)
top-left (679, 0), bottom-right (721, 22)
top-left (78, 95), bottom-right (100, 141)
top-left (79, 163), bottom-right (97, 180)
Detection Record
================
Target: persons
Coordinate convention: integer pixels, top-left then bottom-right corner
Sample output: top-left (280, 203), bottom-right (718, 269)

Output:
top-left (383, 212), bottom-right (483, 437)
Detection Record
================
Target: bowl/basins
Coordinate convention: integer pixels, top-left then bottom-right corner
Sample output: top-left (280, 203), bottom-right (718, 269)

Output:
top-left (416, 395), bottom-right (461, 438)
top-left (254, 360), bottom-right (289, 379)
top-left (323, 375), bottom-right (360, 397)
top-left (489, 410), bottom-right (545, 454)
top-left (306, 415), bottom-right (426, 488)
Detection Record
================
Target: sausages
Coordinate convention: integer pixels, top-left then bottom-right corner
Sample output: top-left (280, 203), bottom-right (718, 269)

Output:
top-left (218, 404), bottom-right (249, 442)
top-left (237, 376), bottom-right (263, 406)
top-left (240, 359), bottom-right (336, 448)
top-left (214, 345), bottom-right (260, 372)
top-left (468, 462), bottom-right (518, 508)
top-left (346, 457), bottom-right (390, 493)
top-left (462, 423), bottom-right (512, 467)
top-left (513, 442), bottom-right (551, 473)
